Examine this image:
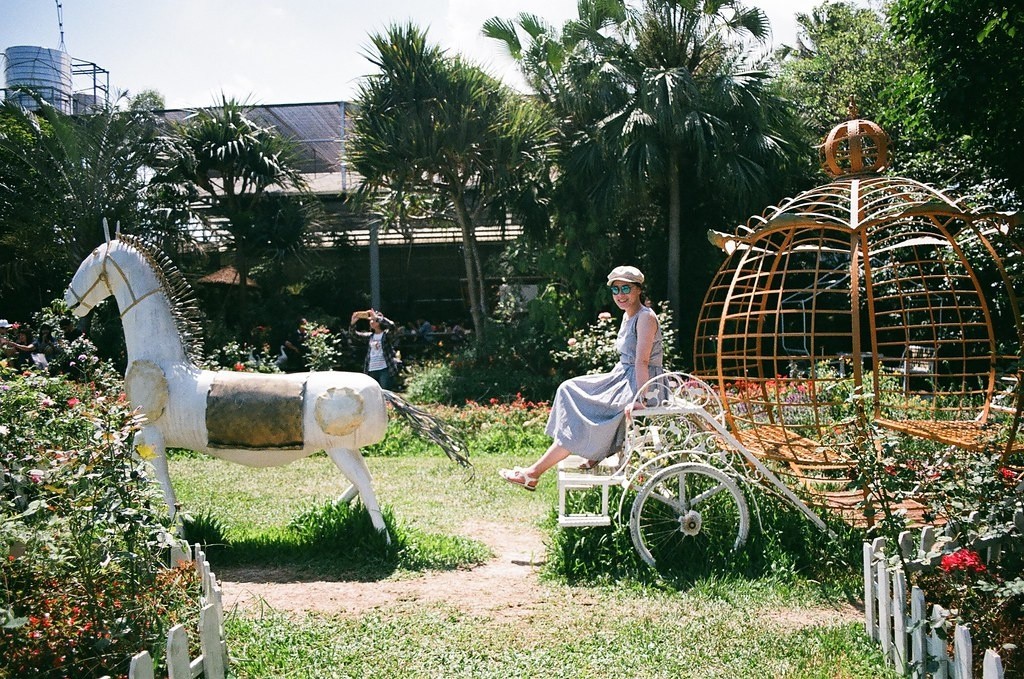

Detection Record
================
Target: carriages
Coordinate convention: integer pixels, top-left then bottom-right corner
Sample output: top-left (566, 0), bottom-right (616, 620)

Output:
top-left (62, 217), bottom-right (840, 579)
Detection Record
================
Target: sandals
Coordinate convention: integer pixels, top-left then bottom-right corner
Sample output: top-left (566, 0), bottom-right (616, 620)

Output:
top-left (498, 466), bottom-right (539, 491)
top-left (577, 458), bottom-right (602, 468)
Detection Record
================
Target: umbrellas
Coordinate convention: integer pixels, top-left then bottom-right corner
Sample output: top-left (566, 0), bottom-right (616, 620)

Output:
top-left (198, 265), bottom-right (261, 290)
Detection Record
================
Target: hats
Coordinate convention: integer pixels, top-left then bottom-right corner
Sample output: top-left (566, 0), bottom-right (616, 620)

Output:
top-left (606, 265), bottom-right (645, 285)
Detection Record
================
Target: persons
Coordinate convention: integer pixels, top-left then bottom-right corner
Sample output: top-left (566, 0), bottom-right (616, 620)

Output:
top-left (285, 318), bottom-right (312, 372)
top-left (500, 266), bottom-right (669, 491)
top-left (398, 316), bottom-right (432, 360)
top-left (447, 319), bottom-right (467, 352)
top-left (348, 308), bottom-right (398, 401)
top-left (0, 318), bottom-right (84, 384)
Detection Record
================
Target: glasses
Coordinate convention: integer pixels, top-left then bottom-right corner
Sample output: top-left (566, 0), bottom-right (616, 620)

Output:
top-left (610, 284), bottom-right (638, 294)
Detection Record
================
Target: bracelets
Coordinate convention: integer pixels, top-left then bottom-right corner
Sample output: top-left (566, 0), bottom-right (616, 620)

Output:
top-left (636, 397), bottom-right (648, 403)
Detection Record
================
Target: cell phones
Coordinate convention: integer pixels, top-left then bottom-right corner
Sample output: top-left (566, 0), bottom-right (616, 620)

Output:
top-left (356, 312), bottom-right (368, 318)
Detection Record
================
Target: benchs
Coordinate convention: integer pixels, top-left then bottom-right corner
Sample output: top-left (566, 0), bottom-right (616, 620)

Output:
top-left (616, 393), bottom-right (700, 472)
top-left (884, 345), bottom-right (939, 397)
top-left (877, 420), bottom-right (1024, 455)
top-left (738, 426), bottom-right (851, 466)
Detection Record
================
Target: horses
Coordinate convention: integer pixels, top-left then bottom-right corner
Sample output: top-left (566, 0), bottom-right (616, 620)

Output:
top-left (62, 217), bottom-right (474, 551)
